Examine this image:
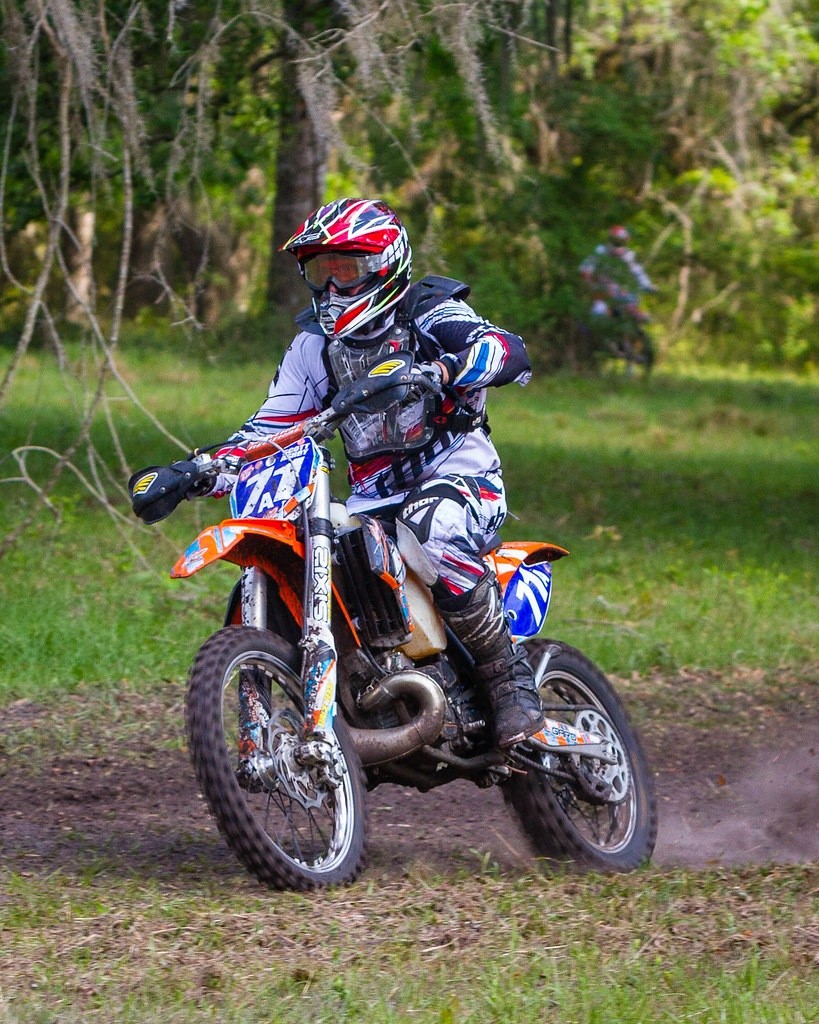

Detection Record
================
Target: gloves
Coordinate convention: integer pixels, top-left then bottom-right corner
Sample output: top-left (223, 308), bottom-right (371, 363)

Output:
top-left (398, 360), bottom-right (443, 408)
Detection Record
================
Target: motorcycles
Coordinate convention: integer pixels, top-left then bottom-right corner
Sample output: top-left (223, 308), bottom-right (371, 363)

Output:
top-left (128, 351), bottom-right (658, 893)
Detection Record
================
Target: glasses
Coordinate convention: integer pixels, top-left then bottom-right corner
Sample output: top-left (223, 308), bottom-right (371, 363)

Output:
top-left (298, 251), bottom-right (381, 293)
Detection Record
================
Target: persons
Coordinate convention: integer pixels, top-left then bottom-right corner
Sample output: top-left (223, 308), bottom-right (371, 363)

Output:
top-left (127, 198), bottom-right (546, 749)
top-left (579, 226), bottom-right (667, 348)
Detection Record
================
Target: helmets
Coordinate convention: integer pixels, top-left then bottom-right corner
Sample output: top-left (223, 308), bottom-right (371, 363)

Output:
top-left (606, 225), bottom-right (630, 255)
top-left (278, 197), bottom-right (414, 339)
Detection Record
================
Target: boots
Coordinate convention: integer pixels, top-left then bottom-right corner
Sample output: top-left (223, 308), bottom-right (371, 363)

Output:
top-left (433, 563), bottom-right (546, 748)
